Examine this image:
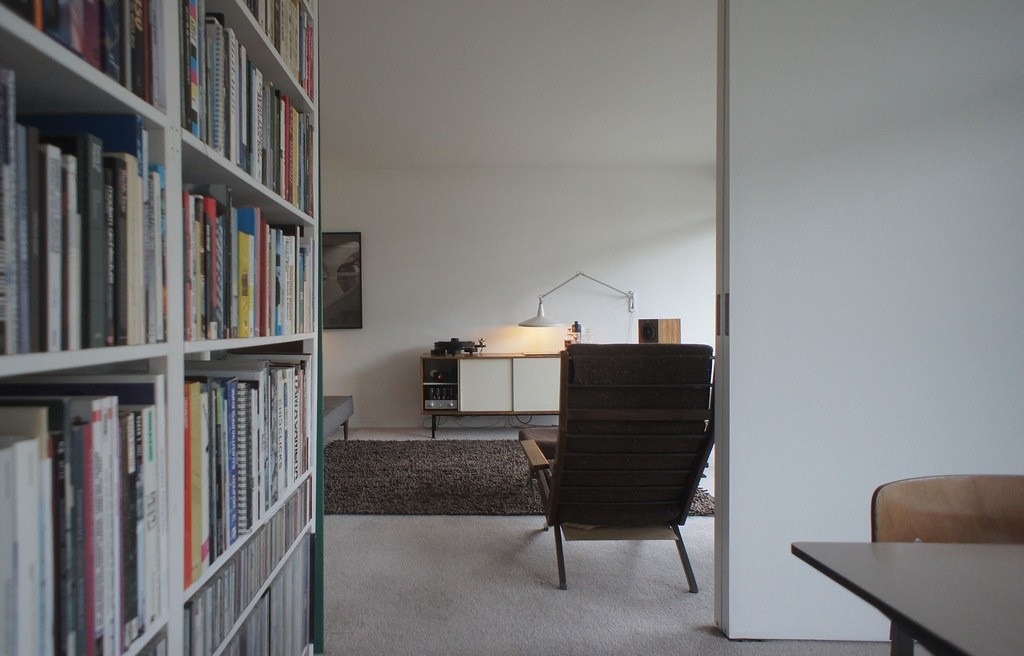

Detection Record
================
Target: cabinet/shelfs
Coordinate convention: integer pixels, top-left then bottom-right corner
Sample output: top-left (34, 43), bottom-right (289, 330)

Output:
top-left (0, 0), bottom-right (325, 656)
top-left (420, 353), bottom-right (561, 439)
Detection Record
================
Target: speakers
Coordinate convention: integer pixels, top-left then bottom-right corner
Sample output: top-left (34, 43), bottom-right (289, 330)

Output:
top-left (638, 318), bottom-right (681, 345)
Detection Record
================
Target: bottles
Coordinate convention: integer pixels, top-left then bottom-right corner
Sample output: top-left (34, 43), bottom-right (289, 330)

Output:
top-left (565, 321), bottom-right (581, 349)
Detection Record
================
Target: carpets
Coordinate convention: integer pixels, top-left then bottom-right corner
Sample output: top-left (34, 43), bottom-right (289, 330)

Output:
top-left (325, 439), bottom-right (717, 517)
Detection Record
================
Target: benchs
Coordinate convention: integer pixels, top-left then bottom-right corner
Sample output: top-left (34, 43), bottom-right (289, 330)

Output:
top-left (322, 395), bottom-right (353, 446)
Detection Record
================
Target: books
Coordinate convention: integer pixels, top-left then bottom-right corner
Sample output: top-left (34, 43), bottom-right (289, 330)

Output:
top-left (0, 0), bottom-right (313, 656)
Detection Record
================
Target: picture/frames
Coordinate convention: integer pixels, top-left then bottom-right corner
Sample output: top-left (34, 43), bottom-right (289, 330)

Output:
top-left (322, 232), bottom-right (363, 330)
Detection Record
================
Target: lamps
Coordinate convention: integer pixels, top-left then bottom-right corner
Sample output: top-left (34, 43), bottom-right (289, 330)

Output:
top-left (519, 271), bottom-right (636, 327)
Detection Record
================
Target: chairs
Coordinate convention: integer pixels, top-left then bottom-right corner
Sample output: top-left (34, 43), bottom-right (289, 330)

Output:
top-left (519, 343), bottom-right (717, 595)
top-left (871, 474), bottom-right (1024, 543)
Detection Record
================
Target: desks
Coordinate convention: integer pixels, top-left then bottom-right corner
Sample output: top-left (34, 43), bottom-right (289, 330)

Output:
top-left (791, 542), bottom-right (1024, 656)
